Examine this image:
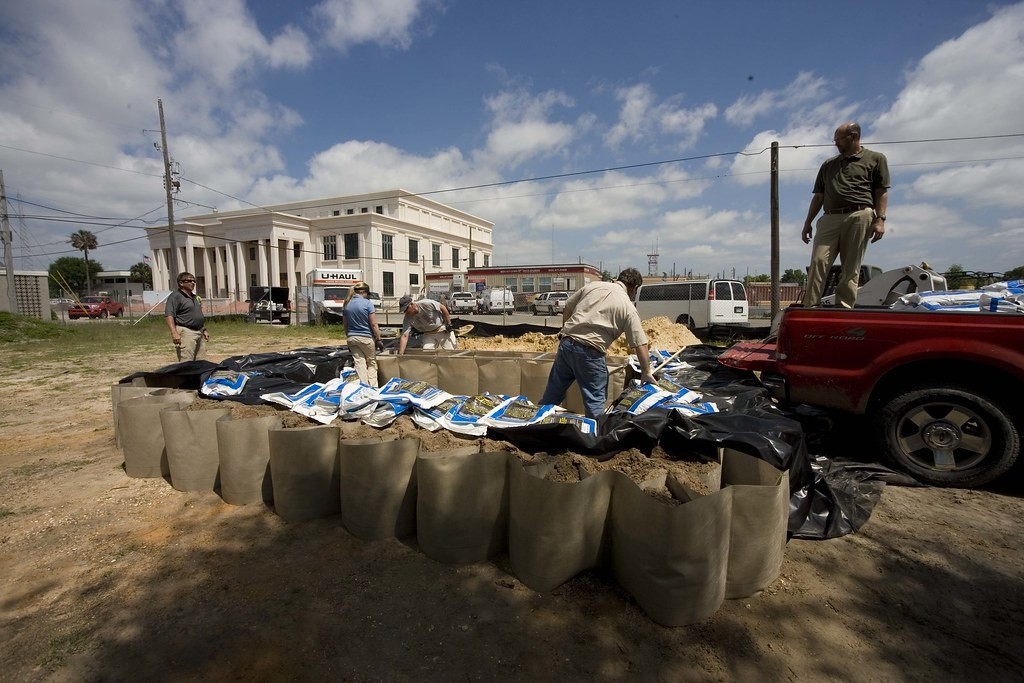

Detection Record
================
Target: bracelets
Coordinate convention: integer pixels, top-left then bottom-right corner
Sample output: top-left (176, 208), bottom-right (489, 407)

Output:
top-left (202, 328), bottom-right (206, 334)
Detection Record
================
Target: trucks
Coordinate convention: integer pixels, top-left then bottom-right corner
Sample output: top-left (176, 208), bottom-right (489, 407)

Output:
top-left (306, 268), bottom-right (365, 326)
top-left (244, 286), bottom-right (292, 325)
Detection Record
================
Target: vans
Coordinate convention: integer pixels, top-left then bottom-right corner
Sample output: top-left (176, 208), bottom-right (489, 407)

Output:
top-left (629, 278), bottom-right (752, 335)
top-left (477, 288), bottom-right (515, 316)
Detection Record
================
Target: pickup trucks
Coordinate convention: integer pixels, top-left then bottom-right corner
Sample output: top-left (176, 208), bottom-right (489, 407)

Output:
top-left (530, 292), bottom-right (570, 316)
top-left (717, 303), bottom-right (1024, 490)
top-left (449, 291), bottom-right (478, 315)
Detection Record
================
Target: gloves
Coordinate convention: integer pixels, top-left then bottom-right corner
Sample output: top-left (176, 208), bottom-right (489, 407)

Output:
top-left (375, 340), bottom-right (385, 352)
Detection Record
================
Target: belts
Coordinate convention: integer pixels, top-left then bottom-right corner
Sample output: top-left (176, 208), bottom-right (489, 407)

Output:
top-left (826, 205), bottom-right (867, 214)
top-left (565, 336), bottom-right (602, 353)
top-left (187, 327), bottom-right (200, 330)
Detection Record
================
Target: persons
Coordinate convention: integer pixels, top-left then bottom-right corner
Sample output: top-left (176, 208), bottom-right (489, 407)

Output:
top-left (538, 268), bottom-right (659, 419)
top-left (165, 272), bottom-right (210, 362)
top-left (802, 121), bottom-right (891, 309)
top-left (399, 294), bottom-right (457, 356)
top-left (343, 281), bottom-right (383, 387)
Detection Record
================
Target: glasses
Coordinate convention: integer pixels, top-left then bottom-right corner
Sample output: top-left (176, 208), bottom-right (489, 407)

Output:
top-left (180, 279), bottom-right (196, 283)
top-left (832, 134), bottom-right (853, 144)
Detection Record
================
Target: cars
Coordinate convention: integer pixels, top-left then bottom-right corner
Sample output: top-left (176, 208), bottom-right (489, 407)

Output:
top-left (369, 292), bottom-right (383, 309)
top-left (68, 295), bottom-right (125, 321)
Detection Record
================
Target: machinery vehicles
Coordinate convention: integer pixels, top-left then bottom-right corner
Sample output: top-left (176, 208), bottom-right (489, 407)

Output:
top-left (763, 262), bottom-right (949, 344)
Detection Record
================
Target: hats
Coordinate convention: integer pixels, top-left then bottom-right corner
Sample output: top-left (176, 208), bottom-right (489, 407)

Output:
top-left (399, 295), bottom-right (412, 312)
top-left (356, 287), bottom-right (371, 295)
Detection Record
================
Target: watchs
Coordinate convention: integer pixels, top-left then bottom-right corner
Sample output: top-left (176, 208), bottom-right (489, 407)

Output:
top-left (877, 214), bottom-right (886, 222)
